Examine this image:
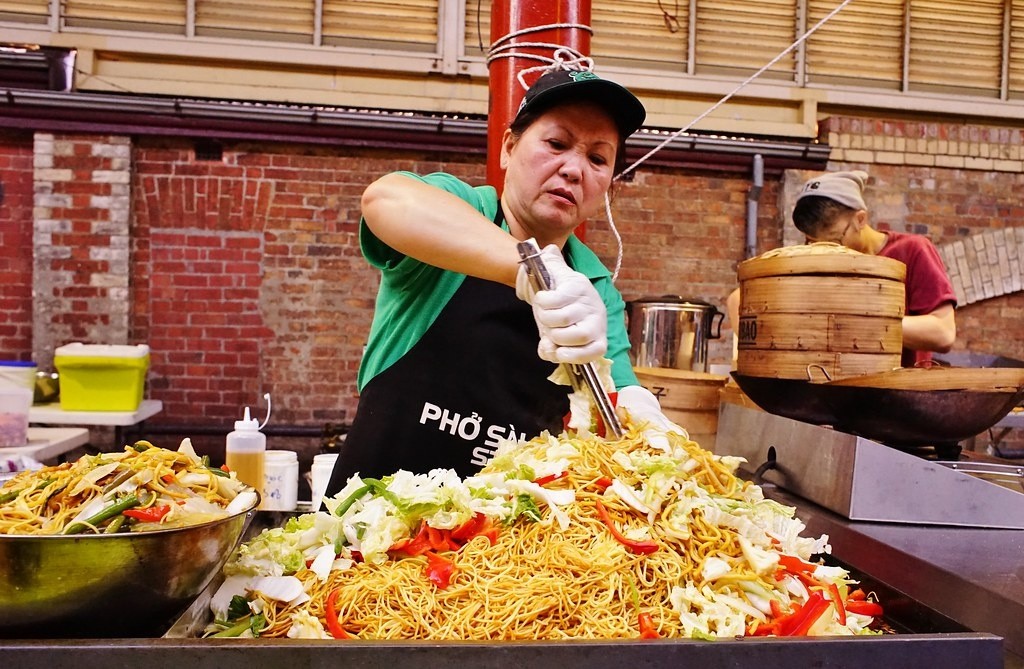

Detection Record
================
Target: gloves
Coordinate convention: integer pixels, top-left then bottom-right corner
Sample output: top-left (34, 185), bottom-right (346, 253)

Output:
top-left (614, 386), bottom-right (685, 437)
top-left (515, 244), bottom-right (607, 364)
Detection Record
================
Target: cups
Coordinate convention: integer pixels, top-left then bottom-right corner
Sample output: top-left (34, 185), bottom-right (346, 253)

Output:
top-left (0, 360), bottom-right (38, 448)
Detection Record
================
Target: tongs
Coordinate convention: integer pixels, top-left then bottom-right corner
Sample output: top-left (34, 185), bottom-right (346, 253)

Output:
top-left (518, 237), bottom-right (624, 442)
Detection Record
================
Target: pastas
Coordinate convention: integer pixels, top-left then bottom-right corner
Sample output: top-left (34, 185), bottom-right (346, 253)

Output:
top-left (0, 440), bottom-right (219, 535)
top-left (248, 417), bottom-right (787, 641)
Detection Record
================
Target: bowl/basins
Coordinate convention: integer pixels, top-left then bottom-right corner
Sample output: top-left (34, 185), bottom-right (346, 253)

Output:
top-left (0, 470), bottom-right (261, 638)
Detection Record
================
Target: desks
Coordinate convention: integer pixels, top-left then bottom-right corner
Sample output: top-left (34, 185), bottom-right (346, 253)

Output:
top-left (0, 427), bottom-right (90, 466)
top-left (28, 402), bottom-right (163, 464)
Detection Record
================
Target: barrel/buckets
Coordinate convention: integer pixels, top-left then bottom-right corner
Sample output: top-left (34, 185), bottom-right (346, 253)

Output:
top-left (623, 292), bottom-right (726, 373)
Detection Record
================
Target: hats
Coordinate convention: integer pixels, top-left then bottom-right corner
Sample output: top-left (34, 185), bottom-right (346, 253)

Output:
top-left (509, 70), bottom-right (646, 161)
top-left (796, 170), bottom-right (868, 211)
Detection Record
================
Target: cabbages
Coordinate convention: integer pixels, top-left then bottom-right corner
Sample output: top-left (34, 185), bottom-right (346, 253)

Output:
top-left (205, 358), bottom-right (883, 638)
top-left (6, 437), bottom-right (257, 532)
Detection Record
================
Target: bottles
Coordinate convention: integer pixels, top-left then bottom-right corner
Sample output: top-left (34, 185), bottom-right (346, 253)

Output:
top-left (263, 449), bottom-right (299, 512)
top-left (224, 407), bottom-right (268, 509)
top-left (310, 453), bottom-right (339, 511)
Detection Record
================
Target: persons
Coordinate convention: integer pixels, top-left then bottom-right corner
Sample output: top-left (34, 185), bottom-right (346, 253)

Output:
top-left (320, 71), bottom-right (691, 512)
top-left (793, 170), bottom-right (957, 369)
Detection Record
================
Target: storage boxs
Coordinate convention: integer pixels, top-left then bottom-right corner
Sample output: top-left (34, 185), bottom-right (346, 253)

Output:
top-left (0, 361), bottom-right (39, 447)
top-left (53, 341), bottom-right (152, 411)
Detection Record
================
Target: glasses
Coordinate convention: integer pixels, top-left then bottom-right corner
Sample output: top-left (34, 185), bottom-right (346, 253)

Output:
top-left (804, 219), bottom-right (853, 246)
